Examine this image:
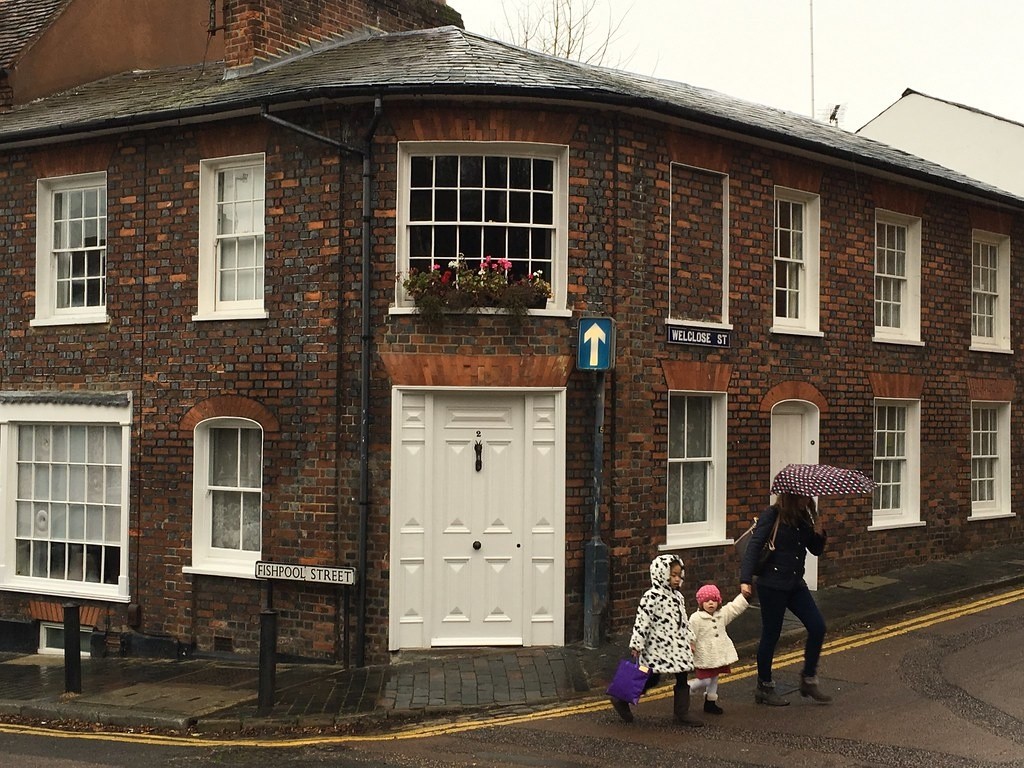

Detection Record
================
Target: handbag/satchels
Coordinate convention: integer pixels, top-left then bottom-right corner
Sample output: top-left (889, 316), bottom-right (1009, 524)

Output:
top-left (735, 505), bottom-right (781, 575)
top-left (605, 655), bottom-right (652, 706)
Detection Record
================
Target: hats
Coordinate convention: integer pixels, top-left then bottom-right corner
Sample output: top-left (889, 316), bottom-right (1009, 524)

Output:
top-left (695, 585), bottom-right (723, 607)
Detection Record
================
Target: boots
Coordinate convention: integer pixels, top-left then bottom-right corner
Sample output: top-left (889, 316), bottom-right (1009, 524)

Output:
top-left (674, 684), bottom-right (704, 727)
top-left (703, 692), bottom-right (724, 714)
top-left (755, 678), bottom-right (791, 707)
top-left (800, 673), bottom-right (832, 702)
top-left (611, 691), bottom-right (647, 722)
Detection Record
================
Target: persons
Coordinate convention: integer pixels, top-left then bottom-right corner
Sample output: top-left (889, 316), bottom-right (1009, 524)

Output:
top-left (608, 554), bottom-right (704, 730)
top-left (686, 584), bottom-right (750, 717)
top-left (738, 491), bottom-right (833, 706)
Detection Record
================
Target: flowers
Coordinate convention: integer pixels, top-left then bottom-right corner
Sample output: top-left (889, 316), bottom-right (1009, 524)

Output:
top-left (402, 252), bottom-right (552, 298)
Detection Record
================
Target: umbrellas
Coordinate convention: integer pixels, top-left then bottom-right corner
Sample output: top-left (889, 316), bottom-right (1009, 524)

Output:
top-left (766, 463), bottom-right (879, 522)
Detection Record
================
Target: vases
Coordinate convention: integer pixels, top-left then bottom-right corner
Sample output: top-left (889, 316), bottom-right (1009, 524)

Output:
top-left (418, 287), bottom-right (539, 306)
